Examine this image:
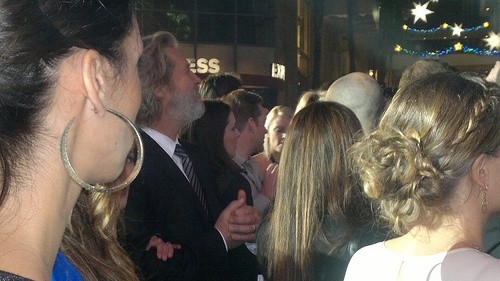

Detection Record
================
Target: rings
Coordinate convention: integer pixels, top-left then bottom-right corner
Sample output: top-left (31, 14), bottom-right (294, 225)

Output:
top-left (252, 224), bottom-right (256, 233)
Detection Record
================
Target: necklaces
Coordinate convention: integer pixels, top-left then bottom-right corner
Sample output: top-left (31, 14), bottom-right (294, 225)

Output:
top-left (439, 228), bottom-right (487, 252)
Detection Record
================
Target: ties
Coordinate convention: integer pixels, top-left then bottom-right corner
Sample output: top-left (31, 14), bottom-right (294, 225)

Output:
top-left (174, 144), bottom-right (208, 214)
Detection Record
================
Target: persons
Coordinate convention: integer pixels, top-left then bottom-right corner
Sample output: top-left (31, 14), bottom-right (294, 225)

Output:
top-left (117, 31), bottom-right (268, 281)
top-left (197, 58), bottom-right (500, 259)
top-left (343, 72), bottom-right (500, 281)
top-left (51, 140), bottom-right (181, 281)
top-left (256, 100), bottom-right (395, 281)
top-left (178, 99), bottom-right (254, 212)
top-left (0, 0), bottom-right (144, 281)
